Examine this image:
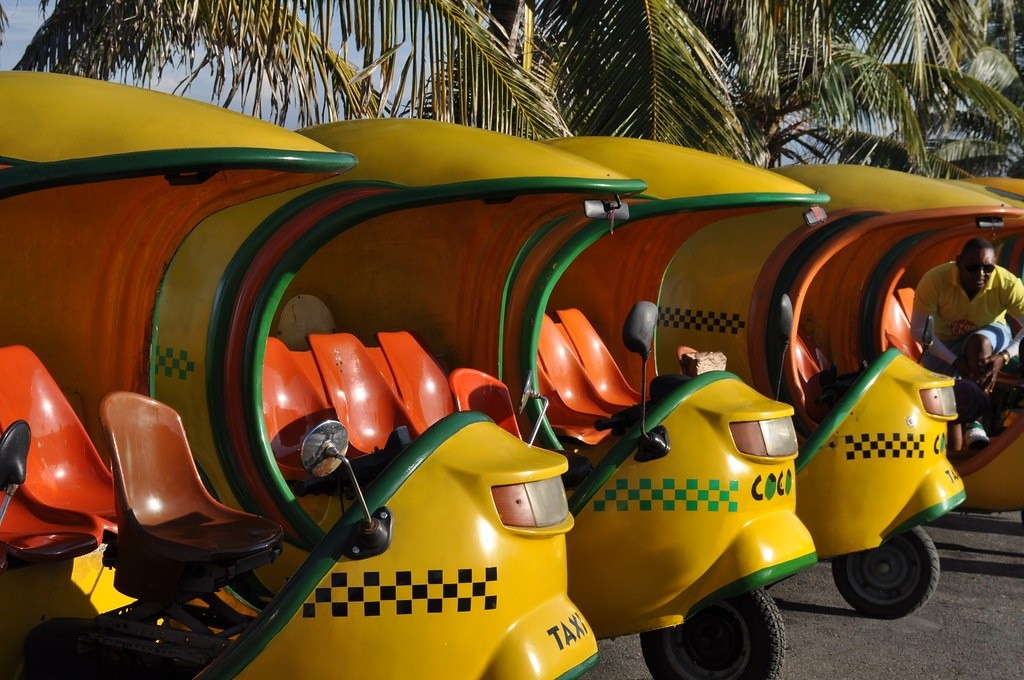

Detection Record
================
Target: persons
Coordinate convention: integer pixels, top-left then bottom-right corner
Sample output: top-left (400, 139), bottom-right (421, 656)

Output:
top-left (911, 237), bottom-right (1024, 448)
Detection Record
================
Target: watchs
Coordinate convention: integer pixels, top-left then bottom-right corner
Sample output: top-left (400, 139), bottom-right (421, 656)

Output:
top-left (999, 352), bottom-right (1009, 365)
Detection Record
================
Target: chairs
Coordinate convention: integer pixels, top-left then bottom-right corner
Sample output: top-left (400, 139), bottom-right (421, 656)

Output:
top-left (0, 346), bottom-right (118, 518)
top-left (539, 313), bottom-right (627, 419)
top-left (0, 484), bottom-right (104, 560)
top-left (263, 337), bottom-right (333, 485)
top-left (308, 332), bottom-right (418, 458)
top-left (377, 331), bottom-right (453, 430)
top-left (447, 368), bottom-right (522, 442)
top-left (897, 287), bottom-right (916, 322)
top-left (885, 294), bottom-right (926, 368)
top-left (793, 336), bottom-right (819, 382)
top-left (535, 361), bottom-right (612, 445)
top-left (101, 391), bottom-right (283, 586)
top-left (556, 308), bottom-right (641, 410)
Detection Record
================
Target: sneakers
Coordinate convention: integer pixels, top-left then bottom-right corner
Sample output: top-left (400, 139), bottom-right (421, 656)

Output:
top-left (963, 420), bottom-right (991, 451)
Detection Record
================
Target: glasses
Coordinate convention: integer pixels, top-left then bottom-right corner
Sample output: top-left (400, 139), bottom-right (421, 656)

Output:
top-left (961, 259), bottom-right (995, 273)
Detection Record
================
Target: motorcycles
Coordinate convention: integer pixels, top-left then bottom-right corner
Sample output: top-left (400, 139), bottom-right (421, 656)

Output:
top-left (1, 66), bottom-right (1023, 680)
